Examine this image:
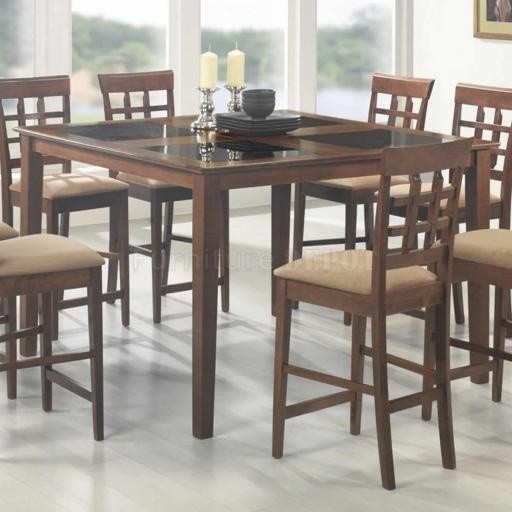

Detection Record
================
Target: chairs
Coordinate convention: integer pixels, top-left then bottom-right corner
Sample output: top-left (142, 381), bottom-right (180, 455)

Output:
top-left (286, 72), bottom-right (436, 327)
top-left (369, 80), bottom-right (508, 326)
top-left (93, 67), bottom-right (232, 323)
top-left (1, 74), bottom-right (134, 328)
top-left (268, 131), bottom-right (477, 491)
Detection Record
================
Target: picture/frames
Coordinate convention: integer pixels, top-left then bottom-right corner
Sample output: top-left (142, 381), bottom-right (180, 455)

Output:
top-left (472, 0), bottom-right (512, 43)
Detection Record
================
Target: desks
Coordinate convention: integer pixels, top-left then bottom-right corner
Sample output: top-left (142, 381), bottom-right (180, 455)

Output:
top-left (12, 106), bottom-right (503, 443)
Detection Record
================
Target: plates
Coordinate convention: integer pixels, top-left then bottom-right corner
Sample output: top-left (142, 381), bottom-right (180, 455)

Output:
top-left (214, 112), bottom-right (302, 136)
top-left (213, 140), bottom-right (296, 152)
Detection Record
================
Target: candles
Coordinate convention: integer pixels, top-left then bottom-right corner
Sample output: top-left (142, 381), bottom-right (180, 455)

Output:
top-left (199, 50), bottom-right (217, 89)
top-left (226, 50), bottom-right (246, 85)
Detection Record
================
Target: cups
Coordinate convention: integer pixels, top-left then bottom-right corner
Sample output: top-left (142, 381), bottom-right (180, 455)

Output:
top-left (242, 88), bottom-right (276, 118)
top-left (241, 153), bottom-right (274, 161)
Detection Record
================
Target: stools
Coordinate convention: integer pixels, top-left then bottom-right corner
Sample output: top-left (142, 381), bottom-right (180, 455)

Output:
top-left (1, 219), bottom-right (20, 403)
top-left (420, 221), bottom-right (512, 421)
top-left (0, 232), bottom-right (115, 442)
